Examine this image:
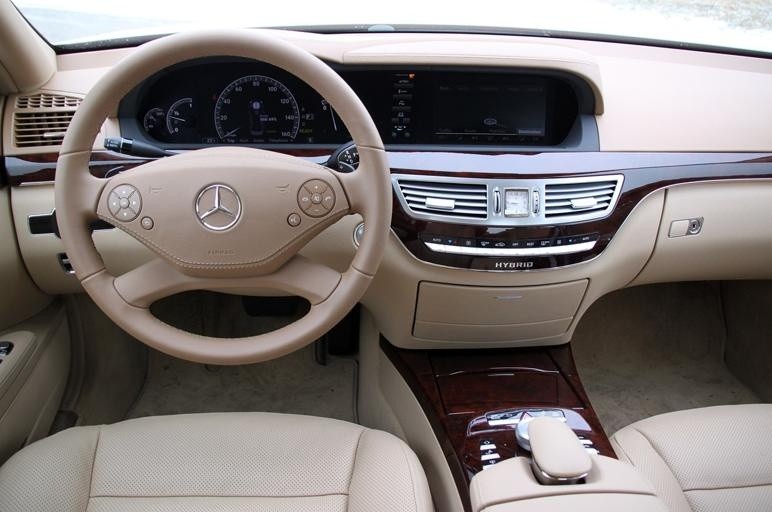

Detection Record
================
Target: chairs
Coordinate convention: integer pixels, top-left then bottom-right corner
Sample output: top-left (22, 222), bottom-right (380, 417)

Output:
top-left (609, 402), bottom-right (772, 512)
top-left (0, 410), bottom-right (435, 512)
